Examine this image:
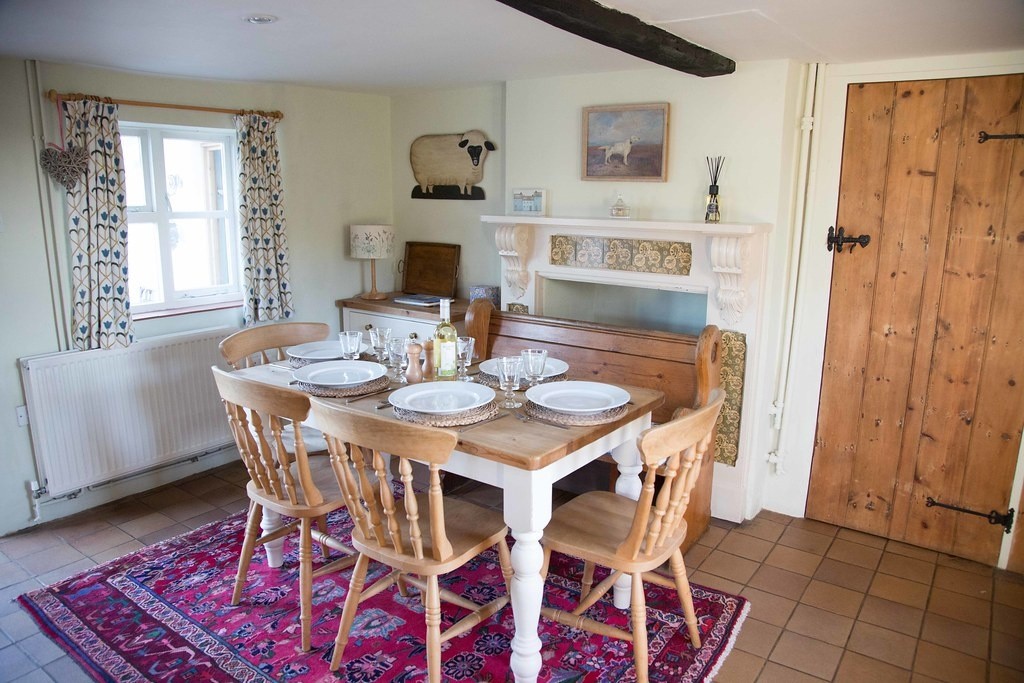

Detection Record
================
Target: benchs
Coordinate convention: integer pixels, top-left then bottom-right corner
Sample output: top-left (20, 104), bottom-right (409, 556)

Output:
top-left (441, 296), bottom-right (723, 565)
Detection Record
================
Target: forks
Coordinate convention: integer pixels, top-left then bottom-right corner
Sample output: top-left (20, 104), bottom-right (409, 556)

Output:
top-left (510, 409), bottom-right (571, 430)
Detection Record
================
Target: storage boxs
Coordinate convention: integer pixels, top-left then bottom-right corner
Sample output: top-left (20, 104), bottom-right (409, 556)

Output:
top-left (469, 285), bottom-right (499, 305)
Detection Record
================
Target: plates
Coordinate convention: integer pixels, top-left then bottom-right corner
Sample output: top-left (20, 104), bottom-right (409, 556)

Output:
top-left (390, 338), bottom-right (465, 359)
top-left (387, 380), bottom-right (496, 414)
top-left (479, 356), bottom-right (570, 378)
top-left (291, 359), bottom-right (388, 389)
top-left (525, 380), bottom-right (631, 415)
top-left (286, 340), bottom-right (368, 359)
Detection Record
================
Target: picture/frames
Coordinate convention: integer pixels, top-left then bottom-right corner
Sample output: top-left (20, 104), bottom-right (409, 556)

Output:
top-left (582, 102), bottom-right (671, 183)
top-left (512, 188), bottom-right (547, 218)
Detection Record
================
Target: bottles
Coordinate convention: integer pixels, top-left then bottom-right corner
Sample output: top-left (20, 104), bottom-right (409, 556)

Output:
top-left (434, 299), bottom-right (457, 379)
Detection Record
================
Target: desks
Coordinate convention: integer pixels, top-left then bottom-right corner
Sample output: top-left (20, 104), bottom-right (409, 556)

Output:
top-left (226, 354), bottom-right (664, 683)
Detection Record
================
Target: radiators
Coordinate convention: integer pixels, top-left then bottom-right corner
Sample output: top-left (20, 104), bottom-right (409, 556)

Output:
top-left (18, 325), bottom-right (240, 521)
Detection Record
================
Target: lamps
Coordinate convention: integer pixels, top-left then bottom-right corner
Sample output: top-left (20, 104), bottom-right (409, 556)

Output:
top-left (349, 224), bottom-right (395, 300)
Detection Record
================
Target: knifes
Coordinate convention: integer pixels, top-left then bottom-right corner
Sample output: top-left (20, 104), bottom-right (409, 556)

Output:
top-left (460, 413), bottom-right (508, 433)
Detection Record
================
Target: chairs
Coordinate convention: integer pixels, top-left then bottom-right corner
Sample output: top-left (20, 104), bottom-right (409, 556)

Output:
top-left (212, 321), bottom-right (726, 683)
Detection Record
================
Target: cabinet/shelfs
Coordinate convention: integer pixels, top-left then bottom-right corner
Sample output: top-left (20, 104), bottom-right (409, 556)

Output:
top-left (341, 291), bottom-right (469, 359)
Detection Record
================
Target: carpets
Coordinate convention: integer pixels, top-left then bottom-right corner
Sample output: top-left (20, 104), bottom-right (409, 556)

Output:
top-left (12, 480), bottom-right (750, 683)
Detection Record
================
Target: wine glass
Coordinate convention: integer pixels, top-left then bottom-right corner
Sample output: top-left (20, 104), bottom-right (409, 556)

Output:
top-left (369, 326), bottom-right (409, 382)
top-left (521, 349), bottom-right (548, 385)
top-left (496, 356), bottom-right (523, 409)
top-left (455, 336), bottom-right (476, 382)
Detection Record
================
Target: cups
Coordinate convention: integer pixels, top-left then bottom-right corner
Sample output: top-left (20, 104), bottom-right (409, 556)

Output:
top-left (338, 331), bottom-right (362, 360)
